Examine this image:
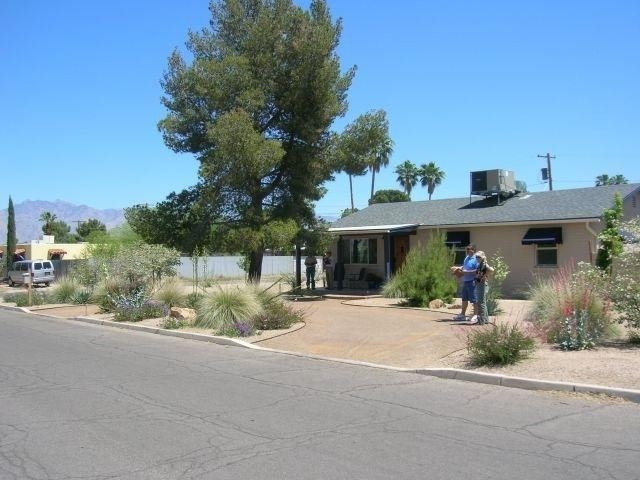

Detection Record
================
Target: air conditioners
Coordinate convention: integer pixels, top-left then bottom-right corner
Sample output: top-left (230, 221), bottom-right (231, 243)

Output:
top-left (470, 169), bottom-right (515, 194)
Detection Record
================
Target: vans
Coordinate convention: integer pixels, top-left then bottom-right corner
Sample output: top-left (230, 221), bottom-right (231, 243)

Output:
top-left (7, 258), bottom-right (55, 289)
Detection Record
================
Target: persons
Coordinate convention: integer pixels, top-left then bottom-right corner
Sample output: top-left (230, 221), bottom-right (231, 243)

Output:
top-left (304, 251), bottom-right (317, 290)
top-left (323, 250), bottom-right (335, 290)
top-left (333, 256), bottom-right (345, 291)
top-left (450, 245), bottom-right (480, 321)
top-left (472, 250), bottom-right (494, 325)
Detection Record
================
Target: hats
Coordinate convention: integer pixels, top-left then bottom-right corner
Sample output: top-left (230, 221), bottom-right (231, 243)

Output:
top-left (475, 251), bottom-right (486, 260)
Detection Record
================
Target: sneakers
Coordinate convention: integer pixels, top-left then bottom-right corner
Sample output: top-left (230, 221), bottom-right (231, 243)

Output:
top-left (453, 314), bottom-right (466, 321)
top-left (468, 314), bottom-right (477, 321)
